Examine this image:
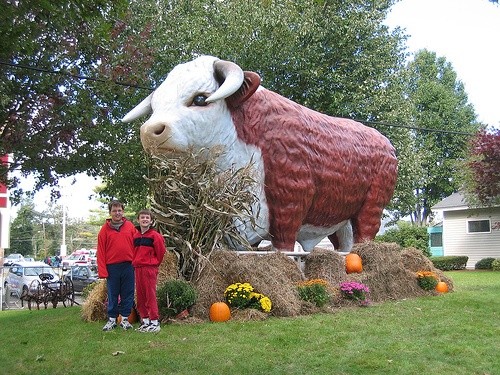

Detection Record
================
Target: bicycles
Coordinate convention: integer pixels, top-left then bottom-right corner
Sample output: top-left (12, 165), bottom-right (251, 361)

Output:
top-left (27, 274), bottom-right (75, 311)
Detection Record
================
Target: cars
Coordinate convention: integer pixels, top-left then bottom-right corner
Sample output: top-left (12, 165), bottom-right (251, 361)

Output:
top-left (5, 262), bottom-right (60, 301)
top-left (50, 249), bottom-right (98, 266)
top-left (4, 253), bottom-right (25, 264)
top-left (64, 263), bottom-right (102, 293)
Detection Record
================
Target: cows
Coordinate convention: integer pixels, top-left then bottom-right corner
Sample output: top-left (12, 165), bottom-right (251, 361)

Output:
top-left (122, 55), bottom-right (398, 252)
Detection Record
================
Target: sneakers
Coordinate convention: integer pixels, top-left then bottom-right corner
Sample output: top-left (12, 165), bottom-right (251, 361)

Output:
top-left (142, 323), bottom-right (160, 333)
top-left (120, 321), bottom-right (133, 331)
top-left (102, 320), bottom-right (117, 330)
top-left (135, 322), bottom-right (149, 330)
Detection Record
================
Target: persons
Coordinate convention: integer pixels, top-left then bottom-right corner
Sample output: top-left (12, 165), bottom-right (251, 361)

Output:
top-left (132, 210), bottom-right (166, 333)
top-left (96, 200), bottom-right (136, 331)
top-left (44, 255), bottom-right (62, 266)
